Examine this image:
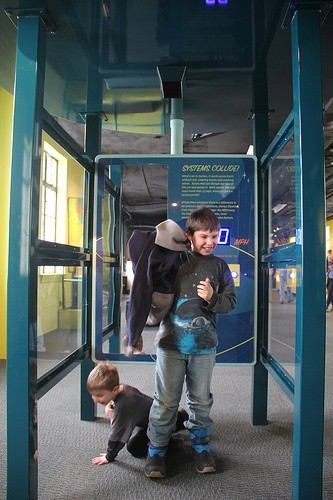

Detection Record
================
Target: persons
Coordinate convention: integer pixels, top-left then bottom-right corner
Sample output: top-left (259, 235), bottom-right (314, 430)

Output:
top-left (279, 249), bottom-right (333, 311)
top-left (143, 207), bottom-right (237, 479)
top-left (87, 362), bottom-right (189, 466)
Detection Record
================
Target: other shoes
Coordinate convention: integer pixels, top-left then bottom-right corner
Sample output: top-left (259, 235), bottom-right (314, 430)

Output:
top-left (144, 447), bottom-right (167, 478)
top-left (190, 444), bottom-right (217, 473)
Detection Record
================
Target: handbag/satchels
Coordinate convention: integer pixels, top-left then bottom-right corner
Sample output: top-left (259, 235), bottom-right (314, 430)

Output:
top-left (169, 298), bottom-right (219, 355)
